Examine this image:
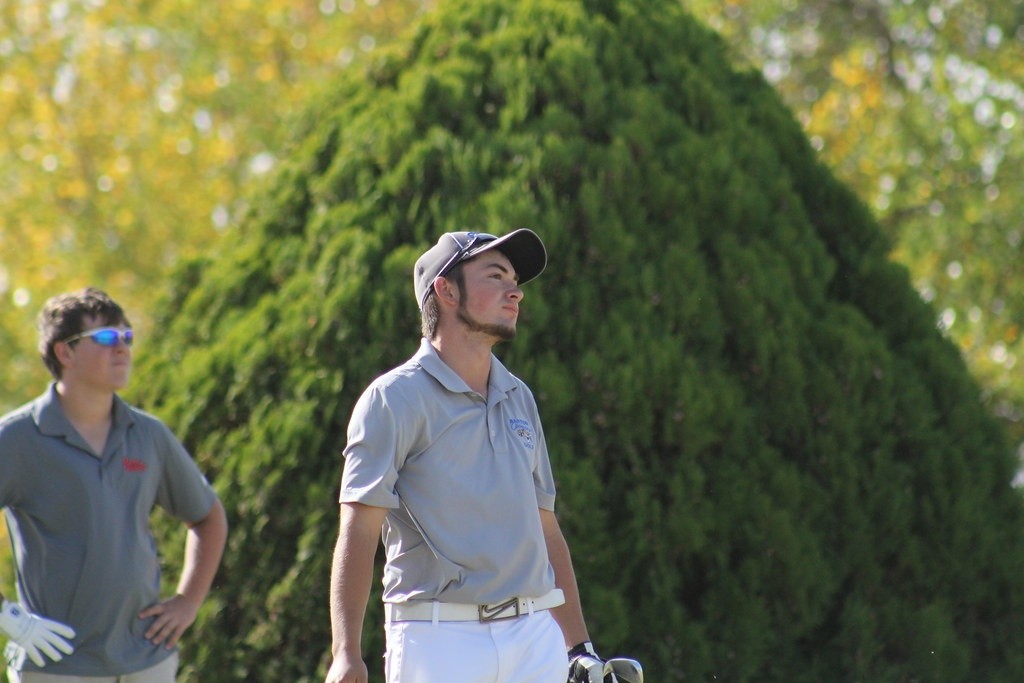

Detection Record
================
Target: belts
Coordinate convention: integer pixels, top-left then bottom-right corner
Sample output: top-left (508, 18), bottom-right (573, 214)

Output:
top-left (383, 586), bottom-right (565, 621)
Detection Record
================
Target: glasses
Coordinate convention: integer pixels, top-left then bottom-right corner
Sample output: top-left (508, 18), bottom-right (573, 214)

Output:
top-left (63, 326), bottom-right (134, 346)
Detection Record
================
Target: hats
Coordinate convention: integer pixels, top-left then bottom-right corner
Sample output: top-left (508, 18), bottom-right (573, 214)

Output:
top-left (412, 228), bottom-right (547, 311)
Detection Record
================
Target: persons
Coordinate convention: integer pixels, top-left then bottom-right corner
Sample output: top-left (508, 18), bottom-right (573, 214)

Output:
top-left (326, 228), bottom-right (619, 683)
top-left (0, 287), bottom-right (228, 683)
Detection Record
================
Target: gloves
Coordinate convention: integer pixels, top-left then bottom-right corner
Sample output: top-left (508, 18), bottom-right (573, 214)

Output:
top-left (0, 601), bottom-right (76, 668)
top-left (568, 640), bottom-right (618, 683)
